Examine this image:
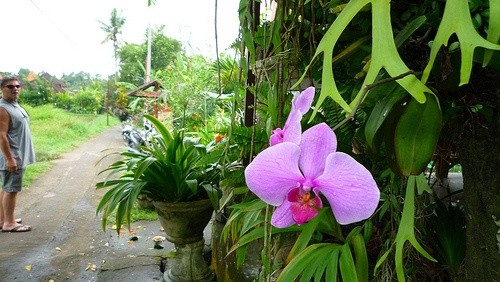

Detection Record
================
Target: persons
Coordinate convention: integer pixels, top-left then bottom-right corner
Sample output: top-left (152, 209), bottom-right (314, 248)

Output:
top-left (0, 74), bottom-right (37, 233)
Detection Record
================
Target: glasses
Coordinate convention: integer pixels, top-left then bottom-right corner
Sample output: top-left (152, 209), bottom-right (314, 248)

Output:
top-left (3, 85), bottom-right (22, 89)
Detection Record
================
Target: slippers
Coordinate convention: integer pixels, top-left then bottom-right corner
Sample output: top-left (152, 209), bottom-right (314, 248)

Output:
top-left (2, 224), bottom-right (31, 231)
top-left (0, 218), bottom-right (22, 229)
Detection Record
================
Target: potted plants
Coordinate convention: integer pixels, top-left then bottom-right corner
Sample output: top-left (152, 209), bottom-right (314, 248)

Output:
top-left (94, 113), bottom-right (236, 241)
